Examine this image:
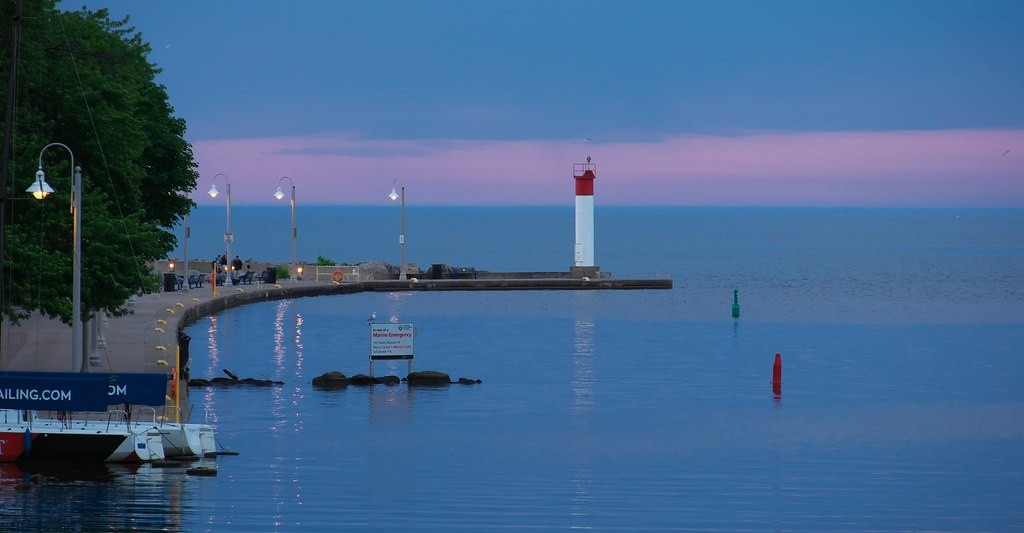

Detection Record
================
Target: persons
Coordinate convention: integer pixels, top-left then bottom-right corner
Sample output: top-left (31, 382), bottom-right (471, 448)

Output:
top-left (232, 255), bottom-right (242, 279)
top-left (216, 255), bottom-right (227, 265)
top-left (237, 265), bottom-right (252, 283)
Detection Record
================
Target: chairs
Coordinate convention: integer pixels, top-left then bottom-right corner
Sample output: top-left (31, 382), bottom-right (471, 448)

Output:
top-left (174, 270), bottom-right (271, 291)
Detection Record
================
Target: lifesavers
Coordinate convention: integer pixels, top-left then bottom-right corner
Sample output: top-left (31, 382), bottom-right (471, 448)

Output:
top-left (333, 271), bottom-right (343, 282)
top-left (210, 272), bottom-right (214, 285)
top-left (170, 368), bottom-right (176, 400)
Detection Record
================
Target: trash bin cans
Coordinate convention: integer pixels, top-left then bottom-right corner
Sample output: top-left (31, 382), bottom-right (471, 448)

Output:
top-left (164, 273), bottom-right (176, 292)
top-left (432, 264), bottom-right (443, 279)
top-left (268, 267), bottom-right (276, 284)
